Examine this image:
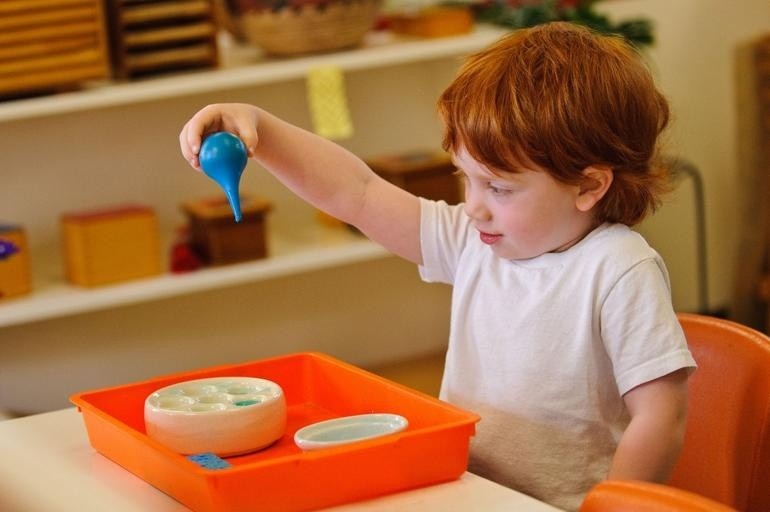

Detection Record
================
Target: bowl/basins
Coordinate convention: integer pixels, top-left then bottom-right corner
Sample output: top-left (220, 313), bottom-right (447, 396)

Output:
top-left (294, 412), bottom-right (410, 452)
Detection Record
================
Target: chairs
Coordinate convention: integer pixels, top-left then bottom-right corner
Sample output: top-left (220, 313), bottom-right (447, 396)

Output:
top-left (579, 480), bottom-right (736, 512)
top-left (641, 312), bottom-right (770, 510)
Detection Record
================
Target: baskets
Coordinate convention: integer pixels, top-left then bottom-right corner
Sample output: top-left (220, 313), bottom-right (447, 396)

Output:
top-left (216, 1), bottom-right (384, 59)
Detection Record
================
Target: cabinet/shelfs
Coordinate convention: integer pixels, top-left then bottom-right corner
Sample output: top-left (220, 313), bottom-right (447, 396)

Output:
top-left (0, 26), bottom-right (511, 327)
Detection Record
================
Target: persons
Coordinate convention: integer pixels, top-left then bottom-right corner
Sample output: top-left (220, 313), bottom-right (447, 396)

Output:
top-left (180, 22), bottom-right (697, 512)
